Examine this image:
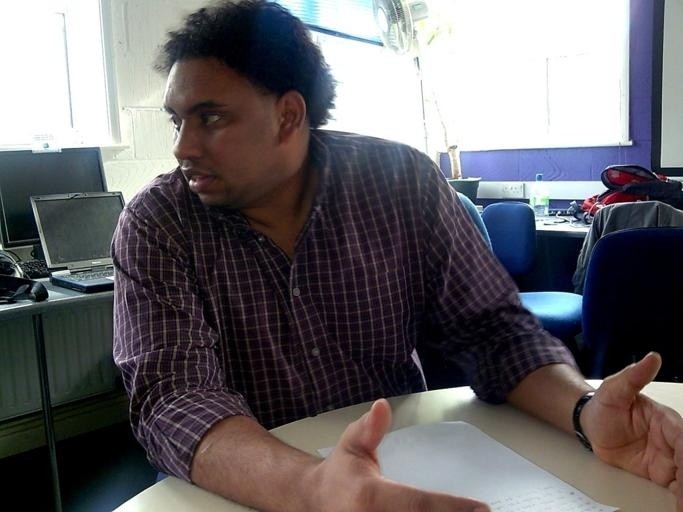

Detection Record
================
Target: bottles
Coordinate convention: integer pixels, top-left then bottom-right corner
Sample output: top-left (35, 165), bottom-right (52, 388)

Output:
top-left (530, 173), bottom-right (549, 221)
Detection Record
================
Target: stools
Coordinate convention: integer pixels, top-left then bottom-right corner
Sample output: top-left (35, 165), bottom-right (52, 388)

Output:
top-left (513, 290), bottom-right (584, 347)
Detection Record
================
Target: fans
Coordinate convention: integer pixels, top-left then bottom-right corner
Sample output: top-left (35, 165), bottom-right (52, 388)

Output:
top-left (372, 0), bottom-right (430, 63)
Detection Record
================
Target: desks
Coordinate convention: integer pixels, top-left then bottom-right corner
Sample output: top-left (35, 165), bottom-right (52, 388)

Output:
top-left (0, 275), bottom-right (128, 512)
top-left (535, 214), bottom-right (589, 238)
top-left (103, 380), bottom-right (683, 512)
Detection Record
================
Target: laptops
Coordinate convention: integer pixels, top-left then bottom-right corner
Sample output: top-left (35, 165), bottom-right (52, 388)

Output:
top-left (29, 191), bottom-right (126, 293)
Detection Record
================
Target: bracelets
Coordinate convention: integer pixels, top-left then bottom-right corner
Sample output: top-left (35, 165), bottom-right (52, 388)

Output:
top-left (572, 390), bottom-right (594, 452)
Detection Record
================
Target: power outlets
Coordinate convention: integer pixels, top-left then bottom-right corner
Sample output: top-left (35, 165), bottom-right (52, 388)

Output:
top-left (503, 183), bottom-right (525, 199)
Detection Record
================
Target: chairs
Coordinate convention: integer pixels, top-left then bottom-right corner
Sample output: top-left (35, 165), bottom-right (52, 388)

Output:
top-left (581, 227), bottom-right (682, 380)
top-left (479, 200), bottom-right (537, 291)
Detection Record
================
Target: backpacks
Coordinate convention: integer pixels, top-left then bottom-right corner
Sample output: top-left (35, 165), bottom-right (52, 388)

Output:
top-left (567, 164), bottom-right (682, 224)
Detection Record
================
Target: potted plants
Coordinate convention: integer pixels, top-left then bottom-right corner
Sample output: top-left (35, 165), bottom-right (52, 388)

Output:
top-left (444, 144), bottom-right (481, 205)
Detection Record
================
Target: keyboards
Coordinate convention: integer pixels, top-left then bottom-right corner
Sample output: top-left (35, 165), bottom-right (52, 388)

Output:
top-left (18, 258), bottom-right (51, 279)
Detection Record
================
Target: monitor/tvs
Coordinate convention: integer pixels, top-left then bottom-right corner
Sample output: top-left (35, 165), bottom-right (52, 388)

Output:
top-left (0, 146), bottom-right (108, 245)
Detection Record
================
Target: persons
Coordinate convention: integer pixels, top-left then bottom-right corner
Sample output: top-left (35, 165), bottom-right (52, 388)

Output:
top-left (111, 0), bottom-right (683, 512)
